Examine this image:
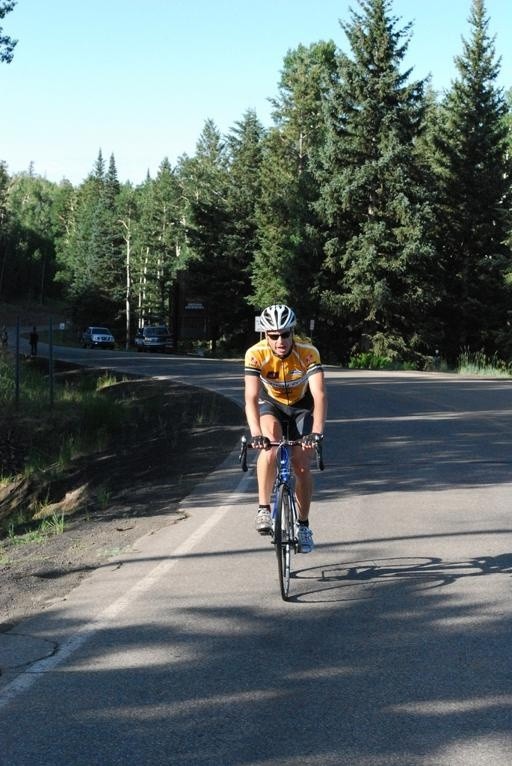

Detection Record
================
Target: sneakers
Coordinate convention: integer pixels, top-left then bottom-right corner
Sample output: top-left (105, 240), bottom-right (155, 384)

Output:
top-left (255, 508), bottom-right (273, 531)
top-left (295, 524), bottom-right (314, 553)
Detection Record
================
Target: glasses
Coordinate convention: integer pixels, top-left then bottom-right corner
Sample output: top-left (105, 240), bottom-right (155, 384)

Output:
top-left (267, 331), bottom-right (291, 340)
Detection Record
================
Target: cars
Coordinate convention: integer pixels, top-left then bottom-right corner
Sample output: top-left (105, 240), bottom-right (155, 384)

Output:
top-left (81, 326), bottom-right (116, 350)
top-left (135, 326), bottom-right (175, 353)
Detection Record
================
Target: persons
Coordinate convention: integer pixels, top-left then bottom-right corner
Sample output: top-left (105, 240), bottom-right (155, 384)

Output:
top-left (0, 321), bottom-right (8, 348)
top-left (29, 326), bottom-right (39, 356)
top-left (244, 305), bottom-right (327, 554)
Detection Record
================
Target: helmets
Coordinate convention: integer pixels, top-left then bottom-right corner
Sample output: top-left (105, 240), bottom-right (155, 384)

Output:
top-left (257, 304), bottom-right (297, 331)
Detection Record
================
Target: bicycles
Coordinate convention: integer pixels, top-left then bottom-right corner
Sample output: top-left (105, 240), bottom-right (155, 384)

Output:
top-left (239, 434), bottom-right (325, 601)
